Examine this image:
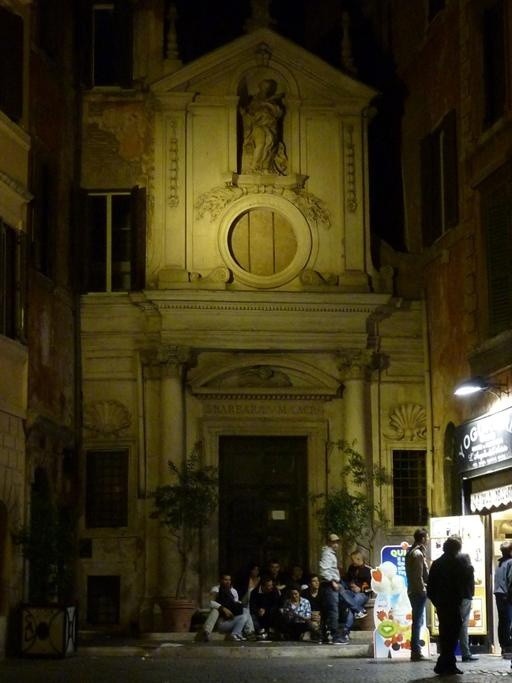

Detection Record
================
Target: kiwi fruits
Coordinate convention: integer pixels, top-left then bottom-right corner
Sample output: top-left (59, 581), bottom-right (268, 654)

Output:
top-left (379, 621), bottom-right (399, 637)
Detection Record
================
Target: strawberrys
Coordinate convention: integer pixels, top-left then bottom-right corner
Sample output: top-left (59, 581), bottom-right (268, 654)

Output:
top-left (375, 609), bottom-right (386, 621)
top-left (371, 569), bottom-right (383, 582)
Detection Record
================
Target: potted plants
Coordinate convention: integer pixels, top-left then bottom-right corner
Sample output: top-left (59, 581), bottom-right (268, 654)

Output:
top-left (145, 442), bottom-right (222, 634)
top-left (307, 439), bottom-right (391, 631)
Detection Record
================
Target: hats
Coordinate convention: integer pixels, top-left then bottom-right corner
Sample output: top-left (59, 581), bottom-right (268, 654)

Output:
top-left (328, 535), bottom-right (339, 543)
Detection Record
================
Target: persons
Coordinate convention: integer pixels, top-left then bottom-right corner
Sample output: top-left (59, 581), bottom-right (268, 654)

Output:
top-left (406, 528), bottom-right (430, 663)
top-left (425, 537), bottom-right (474, 675)
top-left (450, 533), bottom-right (479, 662)
top-left (238, 79), bottom-right (286, 176)
top-left (493, 540), bottom-right (512, 655)
top-left (201, 532), bottom-right (375, 642)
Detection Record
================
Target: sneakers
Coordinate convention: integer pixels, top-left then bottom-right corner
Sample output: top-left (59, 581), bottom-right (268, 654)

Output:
top-left (203, 608), bottom-right (367, 644)
top-left (414, 652), bottom-right (512, 674)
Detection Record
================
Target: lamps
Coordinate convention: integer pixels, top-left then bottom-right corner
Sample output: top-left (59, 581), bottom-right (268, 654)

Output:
top-left (453, 376), bottom-right (507, 396)
